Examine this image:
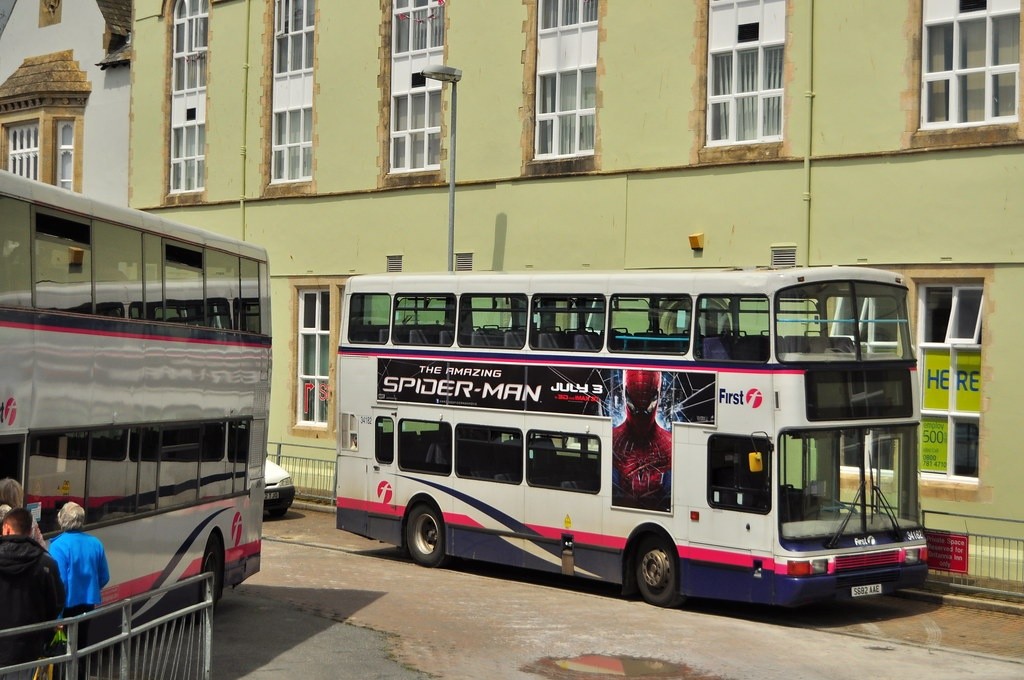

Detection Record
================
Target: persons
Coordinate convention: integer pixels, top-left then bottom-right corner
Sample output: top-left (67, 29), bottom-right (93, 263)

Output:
top-left (48, 501), bottom-right (110, 667)
top-left (0, 478), bottom-right (65, 668)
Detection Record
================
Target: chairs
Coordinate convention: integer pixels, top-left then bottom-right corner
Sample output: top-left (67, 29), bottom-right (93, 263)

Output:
top-left (75, 295), bottom-right (266, 347)
top-left (749, 475), bottom-right (780, 516)
top-left (369, 319), bottom-right (856, 361)
top-left (423, 440), bottom-right (599, 492)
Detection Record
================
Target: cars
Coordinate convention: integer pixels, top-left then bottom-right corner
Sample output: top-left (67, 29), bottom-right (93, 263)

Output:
top-left (263, 452), bottom-right (298, 516)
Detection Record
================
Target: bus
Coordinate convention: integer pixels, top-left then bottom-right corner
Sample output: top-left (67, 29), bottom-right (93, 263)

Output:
top-left (29, 430), bottom-right (247, 517)
top-left (334, 246), bottom-right (929, 610)
top-left (1, 171), bottom-right (276, 638)
